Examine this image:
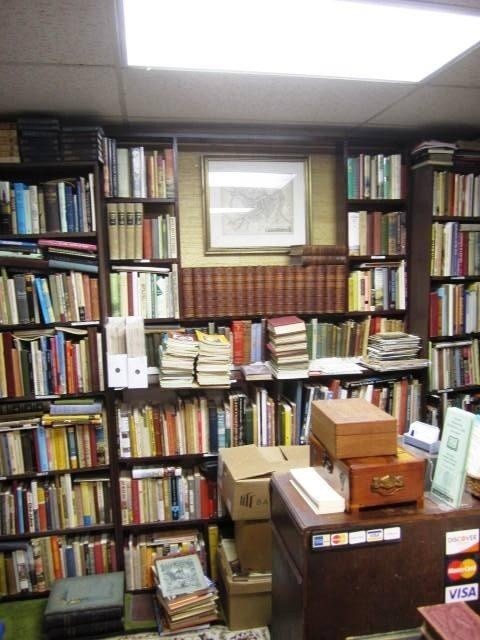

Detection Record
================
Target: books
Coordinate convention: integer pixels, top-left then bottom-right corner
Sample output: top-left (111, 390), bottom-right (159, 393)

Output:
top-left (117, 373), bottom-right (422, 459)
top-left (119, 461), bottom-right (225, 526)
top-left (102, 137), bottom-right (179, 368)
top-left (288, 245), bottom-right (346, 265)
top-left (413, 141), bottom-right (479, 441)
top-left (347, 154), bottom-right (430, 371)
top-left (1, 241), bottom-right (107, 400)
top-left (2, 532), bottom-right (117, 597)
top-left (1, 400), bottom-right (110, 475)
top-left (1, 474), bottom-right (113, 535)
top-left (158, 315), bottom-right (423, 390)
top-left (0, 119), bottom-right (107, 235)
top-left (43, 524), bottom-right (220, 640)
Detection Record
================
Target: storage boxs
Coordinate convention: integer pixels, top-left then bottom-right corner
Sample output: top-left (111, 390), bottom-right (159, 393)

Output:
top-left (210, 545), bottom-right (274, 632)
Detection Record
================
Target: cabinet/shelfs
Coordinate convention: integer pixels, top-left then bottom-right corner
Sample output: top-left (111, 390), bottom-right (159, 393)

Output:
top-left (0, 117), bottom-right (479, 605)
top-left (266, 472), bottom-right (479, 640)
